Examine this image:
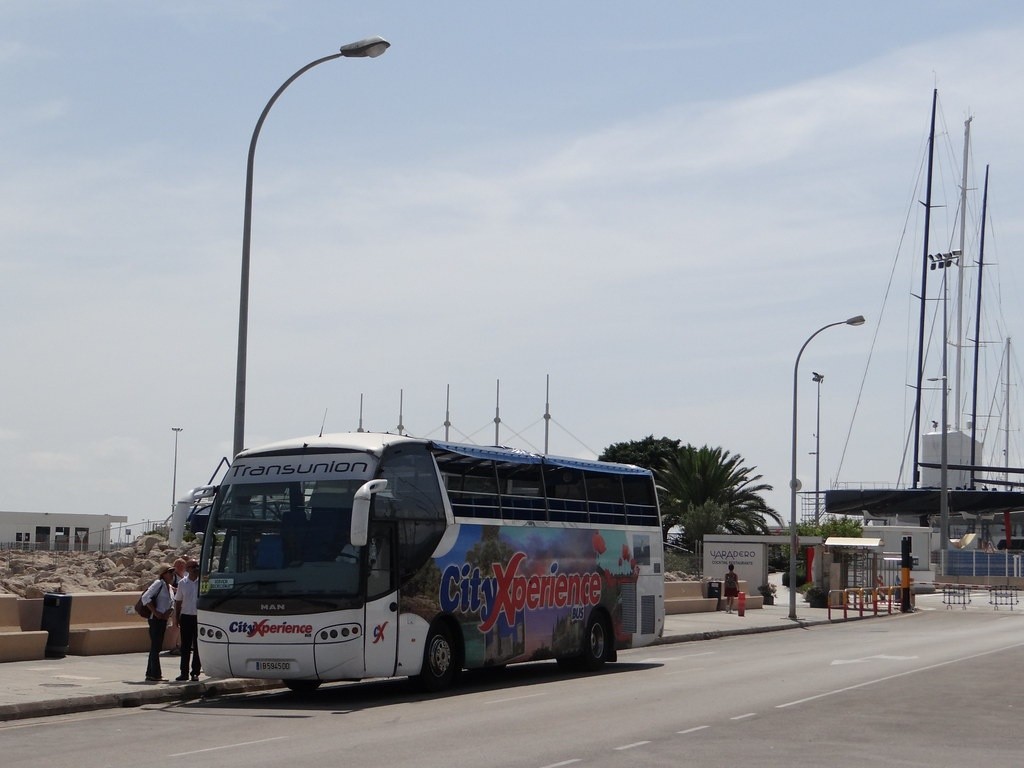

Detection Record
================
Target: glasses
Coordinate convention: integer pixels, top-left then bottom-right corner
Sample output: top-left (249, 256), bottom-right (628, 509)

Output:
top-left (188, 565), bottom-right (200, 568)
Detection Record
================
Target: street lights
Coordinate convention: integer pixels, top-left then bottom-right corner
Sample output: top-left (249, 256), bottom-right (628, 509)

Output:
top-left (928, 249), bottom-right (963, 548)
top-left (811, 371), bottom-right (824, 527)
top-left (171, 427), bottom-right (184, 520)
top-left (235, 38), bottom-right (391, 459)
top-left (789, 315), bottom-right (866, 617)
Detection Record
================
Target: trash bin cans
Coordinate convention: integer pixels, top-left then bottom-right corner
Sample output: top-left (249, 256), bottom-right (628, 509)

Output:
top-left (40, 592), bottom-right (72, 659)
top-left (702, 581), bottom-right (722, 610)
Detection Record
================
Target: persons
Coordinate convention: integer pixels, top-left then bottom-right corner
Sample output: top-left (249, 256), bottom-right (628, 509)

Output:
top-left (170, 558), bottom-right (193, 656)
top-left (173, 560), bottom-right (201, 682)
top-left (725, 564), bottom-right (740, 614)
top-left (142, 565), bottom-right (175, 682)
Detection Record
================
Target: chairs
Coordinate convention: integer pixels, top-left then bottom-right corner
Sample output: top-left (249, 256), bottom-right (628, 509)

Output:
top-left (474, 498), bottom-right (496, 518)
top-left (566, 498), bottom-right (585, 521)
top-left (249, 534), bottom-right (288, 569)
top-left (514, 499), bottom-right (534, 519)
top-left (598, 501), bottom-right (614, 522)
top-left (453, 498), bottom-right (476, 516)
top-left (496, 498), bottom-right (515, 518)
top-left (614, 502), bottom-right (631, 522)
top-left (548, 500), bottom-right (567, 521)
top-left (628, 503), bottom-right (643, 524)
top-left (641, 504), bottom-right (655, 524)
top-left (533, 500), bottom-right (551, 520)
top-left (584, 499), bottom-right (600, 522)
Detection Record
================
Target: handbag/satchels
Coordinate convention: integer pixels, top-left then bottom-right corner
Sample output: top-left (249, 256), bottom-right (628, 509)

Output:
top-left (134, 581), bottom-right (163, 618)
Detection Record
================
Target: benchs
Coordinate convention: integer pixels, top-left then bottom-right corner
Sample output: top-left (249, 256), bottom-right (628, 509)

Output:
top-left (0, 594), bottom-right (49, 662)
top-left (67, 591), bottom-right (181, 656)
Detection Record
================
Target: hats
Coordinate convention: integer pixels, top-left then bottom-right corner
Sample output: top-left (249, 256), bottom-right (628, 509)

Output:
top-left (159, 563), bottom-right (175, 577)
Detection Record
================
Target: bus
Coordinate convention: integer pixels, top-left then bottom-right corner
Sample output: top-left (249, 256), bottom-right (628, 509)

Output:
top-left (184, 431), bottom-right (666, 695)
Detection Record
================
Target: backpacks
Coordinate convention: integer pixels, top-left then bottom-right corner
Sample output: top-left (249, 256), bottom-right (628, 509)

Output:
top-left (726, 574), bottom-right (736, 587)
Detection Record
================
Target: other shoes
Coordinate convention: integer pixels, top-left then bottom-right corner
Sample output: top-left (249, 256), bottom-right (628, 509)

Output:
top-left (192, 674), bottom-right (199, 681)
top-left (176, 674), bottom-right (189, 680)
top-left (726, 605), bottom-right (729, 612)
top-left (146, 675), bottom-right (169, 681)
top-left (171, 648), bottom-right (182, 655)
